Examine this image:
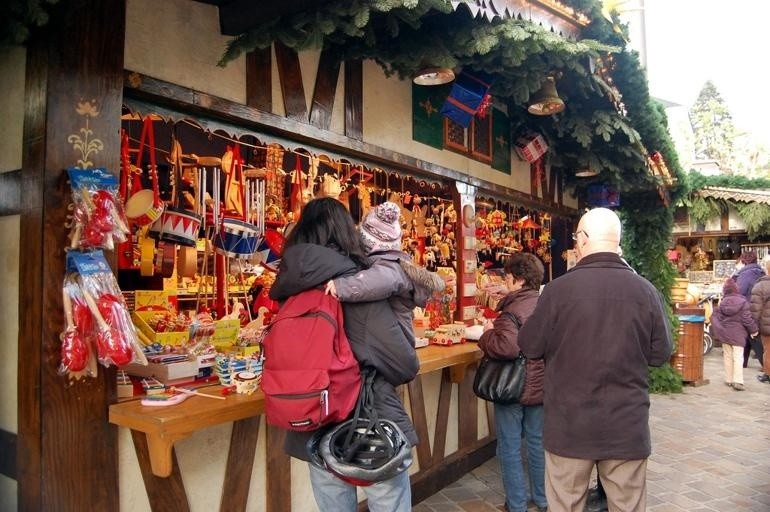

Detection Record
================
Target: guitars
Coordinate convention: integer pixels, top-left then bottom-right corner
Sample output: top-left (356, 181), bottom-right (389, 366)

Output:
top-left (119, 161), bottom-right (197, 277)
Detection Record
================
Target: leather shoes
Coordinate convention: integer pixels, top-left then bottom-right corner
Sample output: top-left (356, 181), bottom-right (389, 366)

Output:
top-left (758, 374), bottom-right (770, 383)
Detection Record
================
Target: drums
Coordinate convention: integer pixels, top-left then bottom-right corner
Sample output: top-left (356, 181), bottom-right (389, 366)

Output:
top-left (249, 228), bottom-right (287, 272)
top-left (212, 217), bottom-right (259, 259)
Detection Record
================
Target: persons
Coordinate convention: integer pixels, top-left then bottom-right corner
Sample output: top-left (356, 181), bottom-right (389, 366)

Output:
top-left (709, 245), bottom-right (770, 391)
top-left (323, 200), bottom-right (445, 447)
top-left (269, 195), bottom-right (421, 511)
top-left (475, 252), bottom-right (548, 511)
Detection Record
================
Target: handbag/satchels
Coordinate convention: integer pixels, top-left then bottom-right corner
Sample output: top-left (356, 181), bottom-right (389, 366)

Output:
top-left (473, 354), bottom-right (527, 404)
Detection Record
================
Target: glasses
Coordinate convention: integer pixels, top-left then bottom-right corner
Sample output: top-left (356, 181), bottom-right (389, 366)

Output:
top-left (571, 230), bottom-right (589, 241)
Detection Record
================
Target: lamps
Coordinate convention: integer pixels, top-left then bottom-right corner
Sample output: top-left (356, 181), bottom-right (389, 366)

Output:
top-left (527, 70), bottom-right (566, 116)
top-left (573, 144), bottom-right (600, 178)
top-left (413, 66), bottom-right (456, 86)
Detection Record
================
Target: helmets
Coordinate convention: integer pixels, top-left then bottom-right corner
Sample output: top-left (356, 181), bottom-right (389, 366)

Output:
top-left (306, 417), bottom-right (413, 488)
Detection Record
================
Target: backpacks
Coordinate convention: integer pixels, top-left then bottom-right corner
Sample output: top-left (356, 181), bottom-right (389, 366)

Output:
top-left (261, 279), bottom-right (372, 432)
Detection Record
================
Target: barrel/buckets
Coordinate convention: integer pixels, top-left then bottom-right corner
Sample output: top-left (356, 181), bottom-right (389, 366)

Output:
top-left (671, 278), bottom-right (688, 302)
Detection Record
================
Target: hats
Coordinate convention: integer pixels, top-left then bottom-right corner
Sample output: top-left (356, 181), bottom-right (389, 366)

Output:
top-left (723, 278), bottom-right (740, 294)
top-left (357, 201), bottom-right (402, 255)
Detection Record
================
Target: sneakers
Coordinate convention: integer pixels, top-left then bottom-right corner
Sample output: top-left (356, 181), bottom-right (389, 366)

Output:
top-left (726, 382), bottom-right (746, 390)
top-left (586, 487), bottom-right (607, 511)
